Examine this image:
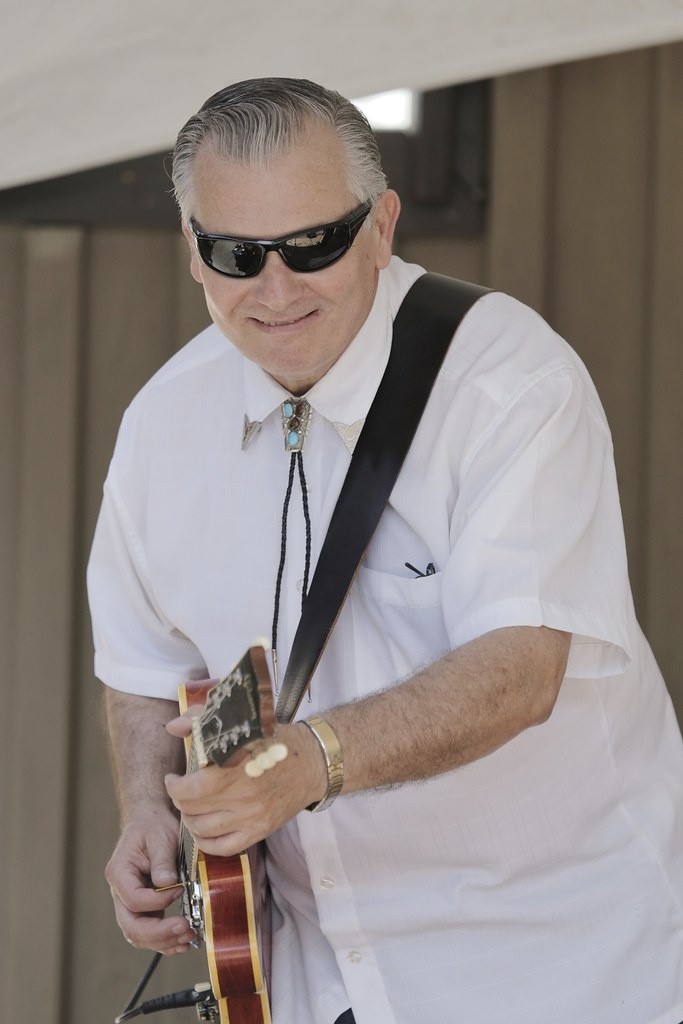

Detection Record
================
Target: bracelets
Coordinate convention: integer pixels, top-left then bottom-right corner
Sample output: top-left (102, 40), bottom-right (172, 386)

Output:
top-left (295, 714), bottom-right (343, 813)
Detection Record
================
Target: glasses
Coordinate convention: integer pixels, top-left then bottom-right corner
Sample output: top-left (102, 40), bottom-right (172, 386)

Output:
top-left (190, 201), bottom-right (373, 278)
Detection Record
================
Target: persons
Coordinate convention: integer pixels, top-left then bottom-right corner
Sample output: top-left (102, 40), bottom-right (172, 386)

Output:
top-left (86, 77), bottom-right (683, 1024)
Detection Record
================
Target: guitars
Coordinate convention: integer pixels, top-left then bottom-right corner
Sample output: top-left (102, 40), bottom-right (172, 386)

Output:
top-left (175, 643), bottom-right (277, 1023)
